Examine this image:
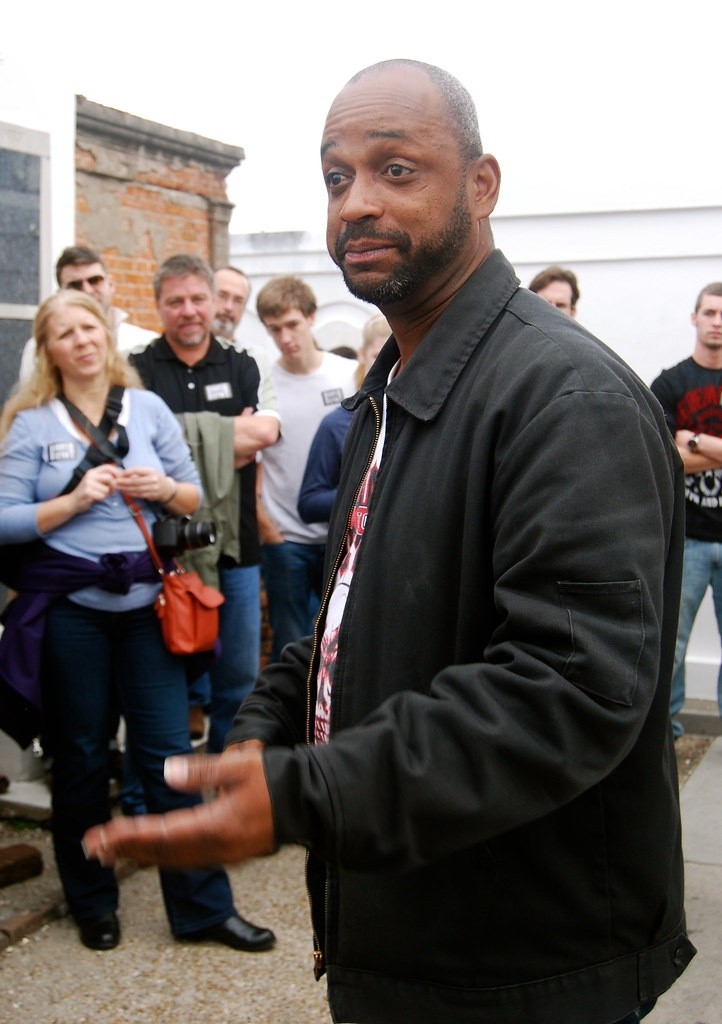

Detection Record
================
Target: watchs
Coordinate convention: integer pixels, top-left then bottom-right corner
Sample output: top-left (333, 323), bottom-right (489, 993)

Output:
top-left (688, 432), bottom-right (702, 453)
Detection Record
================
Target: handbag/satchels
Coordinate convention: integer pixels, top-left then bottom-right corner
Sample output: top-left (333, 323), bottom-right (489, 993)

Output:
top-left (152, 559), bottom-right (225, 655)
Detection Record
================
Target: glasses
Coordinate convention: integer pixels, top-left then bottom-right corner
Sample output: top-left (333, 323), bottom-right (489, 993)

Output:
top-left (66, 275), bottom-right (105, 290)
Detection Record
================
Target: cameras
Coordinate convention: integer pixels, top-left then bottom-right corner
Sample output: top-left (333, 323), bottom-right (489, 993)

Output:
top-left (151, 511), bottom-right (219, 556)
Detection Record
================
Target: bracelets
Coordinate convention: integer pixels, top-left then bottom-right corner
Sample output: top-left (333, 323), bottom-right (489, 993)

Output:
top-left (160, 476), bottom-right (179, 507)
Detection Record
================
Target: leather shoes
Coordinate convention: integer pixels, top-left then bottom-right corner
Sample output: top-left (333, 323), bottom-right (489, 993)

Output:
top-left (176, 914), bottom-right (278, 952)
top-left (77, 912), bottom-right (120, 950)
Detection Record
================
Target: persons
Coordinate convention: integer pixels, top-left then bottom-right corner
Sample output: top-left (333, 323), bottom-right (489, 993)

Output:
top-left (84, 59), bottom-right (701, 1024)
top-left (0, 244), bottom-right (393, 952)
top-left (526, 264), bottom-right (722, 742)
